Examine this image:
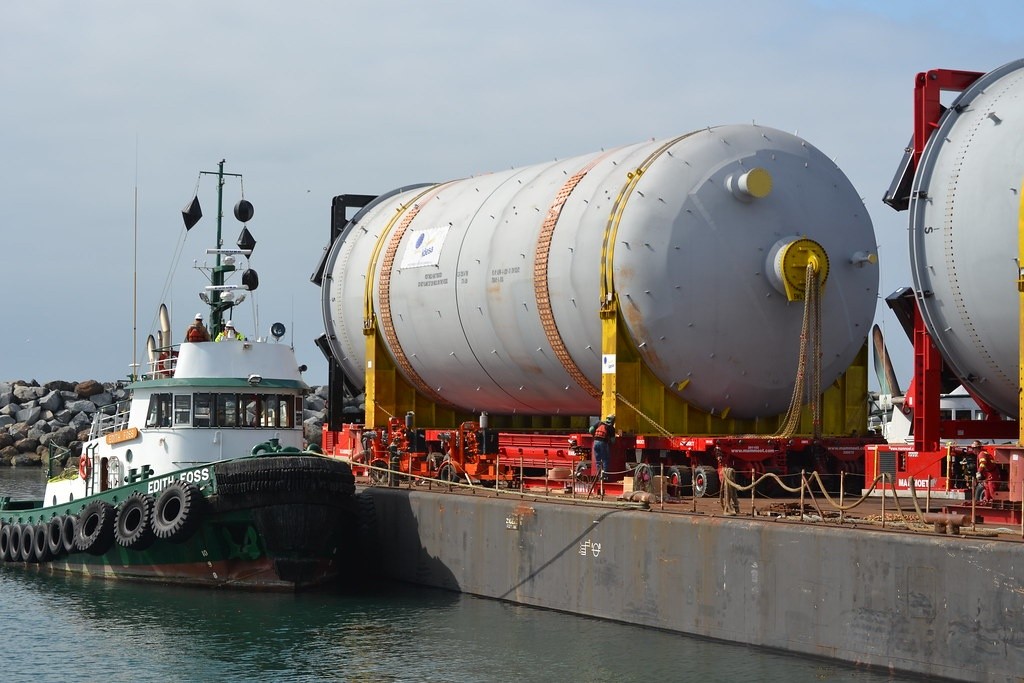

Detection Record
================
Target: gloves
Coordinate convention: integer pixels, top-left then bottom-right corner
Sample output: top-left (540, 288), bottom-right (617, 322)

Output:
top-left (976, 472), bottom-right (983, 479)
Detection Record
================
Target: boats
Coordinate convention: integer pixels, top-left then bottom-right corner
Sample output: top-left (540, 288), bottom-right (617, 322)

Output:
top-left (0, 158), bottom-right (359, 593)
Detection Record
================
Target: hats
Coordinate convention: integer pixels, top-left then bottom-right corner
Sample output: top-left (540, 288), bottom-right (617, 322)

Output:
top-left (971, 441), bottom-right (984, 448)
top-left (606, 414), bottom-right (615, 423)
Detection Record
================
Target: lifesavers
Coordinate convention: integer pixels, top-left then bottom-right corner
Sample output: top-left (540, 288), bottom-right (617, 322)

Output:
top-left (74, 500), bottom-right (116, 557)
top-left (0, 524), bottom-right (13, 562)
top-left (34, 523), bottom-right (55, 563)
top-left (113, 491), bottom-right (156, 550)
top-left (150, 479), bottom-right (204, 541)
top-left (21, 523), bottom-right (37, 564)
top-left (159, 351), bottom-right (179, 376)
top-left (48, 516), bottom-right (67, 556)
top-left (10, 522), bottom-right (26, 563)
top-left (63, 513), bottom-right (82, 555)
top-left (80, 454), bottom-right (91, 480)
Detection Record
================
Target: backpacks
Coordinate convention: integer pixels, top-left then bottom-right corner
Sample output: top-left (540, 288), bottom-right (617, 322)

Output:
top-left (594, 424), bottom-right (607, 439)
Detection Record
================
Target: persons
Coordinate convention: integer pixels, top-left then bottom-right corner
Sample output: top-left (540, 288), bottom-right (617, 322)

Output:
top-left (215, 320), bottom-right (243, 342)
top-left (389, 436), bottom-right (403, 486)
top-left (185, 313), bottom-right (212, 342)
top-left (970, 440), bottom-right (1001, 502)
top-left (588, 414), bottom-right (616, 482)
top-left (875, 429), bottom-right (887, 444)
